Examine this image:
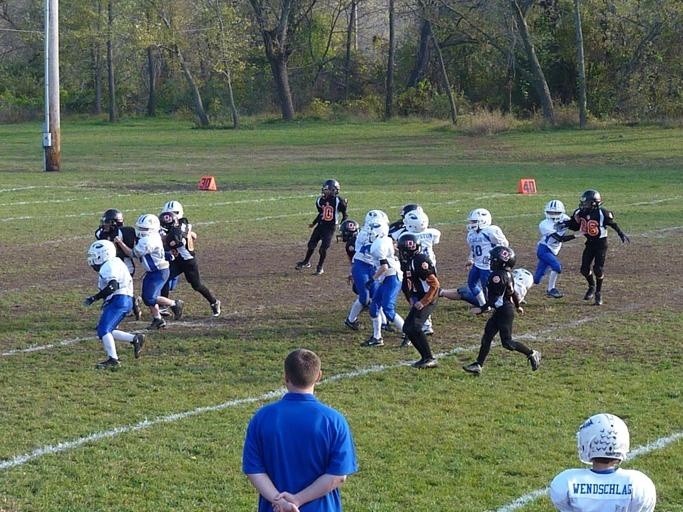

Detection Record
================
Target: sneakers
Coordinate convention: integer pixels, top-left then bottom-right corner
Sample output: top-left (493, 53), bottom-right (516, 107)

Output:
top-left (584, 288), bottom-right (603, 306)
top-left (412, 357), bottom-right (438, 367)
top-left (96, 332), bottom-right (143, 368)
top-left (134, 296), bottom-right (185, 329)
top-left (463, 362), bottom-right (482, 373)
top-left (529, 350), bottom-right (541, 370)
top-left (547, 288), bottom-right (562, 298)
top-left (211, 299), bottom-right (220, 315)
top-left (345, 317), bottom-right (433, 347)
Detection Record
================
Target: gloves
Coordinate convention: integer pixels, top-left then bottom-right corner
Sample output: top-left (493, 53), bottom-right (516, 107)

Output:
top-left (620, 231), bottom-right (625, 243)
top-left (83, 296), bottom-right (94, 306)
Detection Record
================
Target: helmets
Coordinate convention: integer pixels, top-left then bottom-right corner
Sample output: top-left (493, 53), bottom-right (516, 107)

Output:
top-left (544, 189), bottom-right (601, 221)
top-left (340, 203), bottom-right (428, 258)
top-left (577, 414), bottom-right (630, 464)
top-left (466, 208), bottom-right (492, 231)
top-left (489, 247), bottom-right (534, 289)
top-left (322, 180), bottom-right (340, 196)
top-left (87, 201), bottom-right (184, 272)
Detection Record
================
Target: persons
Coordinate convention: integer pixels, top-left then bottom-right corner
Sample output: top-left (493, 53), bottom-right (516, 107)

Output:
top-left (241, 350), bottom-right (358, 512)
top-left (569, 190), bottom-right (630, 305)
top-left (459, 247), bottom-right (542, 376)
top-left (340, 204), bottom-right (442, 346)
top-left (440, 268), bottom-right (534, 306)
top-left (296, 179), bottom-right (348, 275)
top-left (96, 201), bottom-right (221, 330)
top-left (548, 413), bottom-right (658, 511)
top-left (534, 200), bottom-right (587, 297)
top-left (82, 238), bottom-right (147, 369)
top-left (397, 235), bottom-right (442, 369)
top-left (468, 207), bottom-right (512, 316)
